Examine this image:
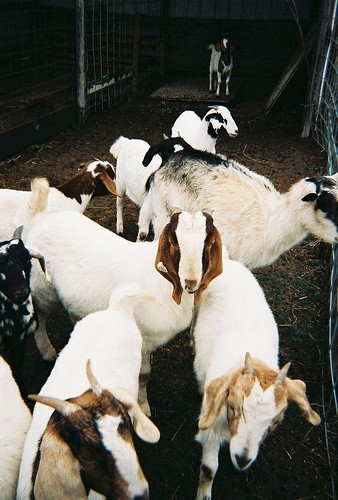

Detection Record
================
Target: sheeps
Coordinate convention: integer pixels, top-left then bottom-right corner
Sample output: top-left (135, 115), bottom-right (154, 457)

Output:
top-left (0, 223), bottom-right (58, 362)
top-left (14, 279), bottom-right (163, 500)
top-left (108, 131), bottom-right (338, 271)
top-left (170, 104), bottom-right (239, 155)
top-left (18, 207), bottom-right (224, 416)
top-left (0, 155), bottom-right (120, 239)
top-left (207, 37), bottom-right (234, 95)
top-left (189, 241), bottom-right (321, 499)
